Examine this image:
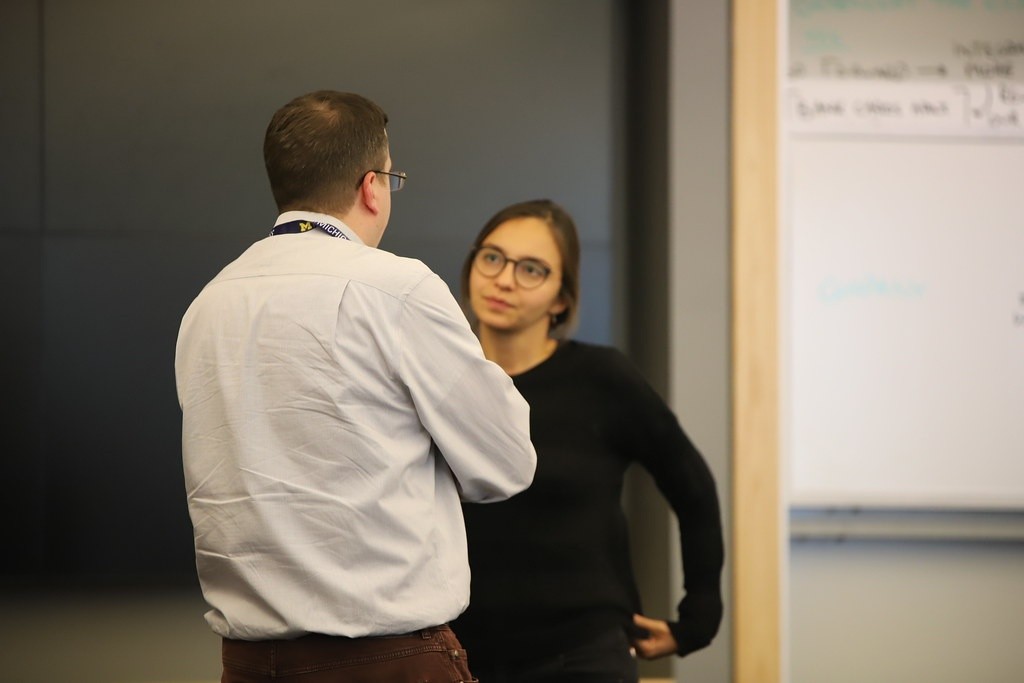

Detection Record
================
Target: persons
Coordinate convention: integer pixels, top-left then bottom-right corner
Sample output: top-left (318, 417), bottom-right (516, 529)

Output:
top-left (175, 90), bottom-right (536, 683)
top-left (451, 199), bottom-right (724, 683)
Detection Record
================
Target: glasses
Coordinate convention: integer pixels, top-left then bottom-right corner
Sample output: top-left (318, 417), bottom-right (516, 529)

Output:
top-left (470, 243), bottom-right (560, 290)
top-left (355, 169), bottom-right (407, 191)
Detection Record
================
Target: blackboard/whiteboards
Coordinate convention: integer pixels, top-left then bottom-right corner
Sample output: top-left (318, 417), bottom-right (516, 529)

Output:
top-left (783, 76), bottom-right (1024, 543)
